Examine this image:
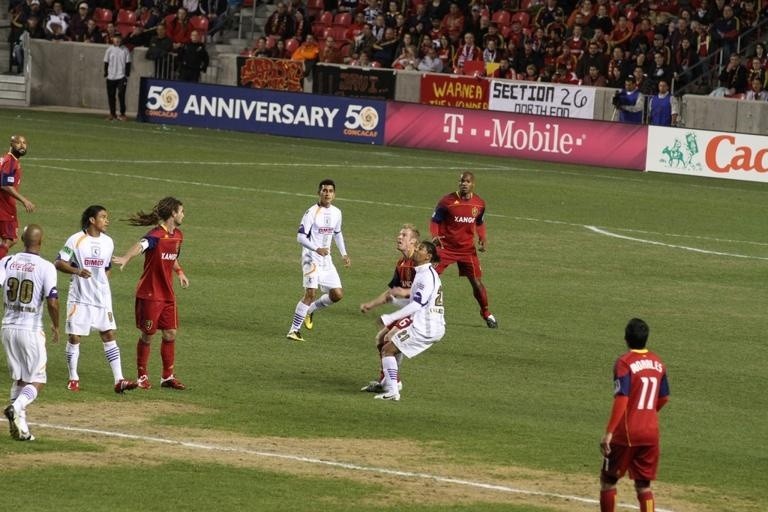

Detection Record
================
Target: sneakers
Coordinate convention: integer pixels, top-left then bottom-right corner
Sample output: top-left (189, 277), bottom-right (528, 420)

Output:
top-left (117, 115), bottom-right (128, 121)
top-left (114, 379), bottom-right (138, 394)
top-left (16, 430), bottom-right (36, 440)
top-left (360, 383), bottom-right (383, 393)
top-left (104, 116), bottom-right (117, 122)
top-left (160, 377), bottom-right (186, 390)
top-left (137, 375), bottom-right (151, 389)
top-left (67, 379), bottom-right (80, 392)
top-left (373, 392), bottom-right (401, 401)
top-left (303, 310), bottom-right (314, 330)
top-left (479, 309), bottom-right (497, 328)
top-left (286, 330), bottom-right (306, 341)
top-left (4, 404), bottom-right (21, 438)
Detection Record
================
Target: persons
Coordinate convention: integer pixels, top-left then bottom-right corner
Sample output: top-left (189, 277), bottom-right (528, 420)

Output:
top-left (111, 196), bottom-right (189, 390)
top-left (286, 179), bottom-right (350, 341)
top-left (599, 317), bottom-right (670, 512)
top-left (360, 223), bottom-right (420, 392)
top-left (53, 205), bottom-right (140, 394)
top-left (430, 172), bottom-right (497, 329)
top-left (0, 223), bottom-right (60, 440)
top-left (374, 240), bottom-right (446, 401)
top-left (0, 135), bottom-right (35, 260)
top-left (7, 1), bottom-right (767, 131)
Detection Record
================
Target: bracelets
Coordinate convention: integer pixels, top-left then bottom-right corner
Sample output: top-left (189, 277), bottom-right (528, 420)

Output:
top-left (175, 268), bottom-right (184, 275)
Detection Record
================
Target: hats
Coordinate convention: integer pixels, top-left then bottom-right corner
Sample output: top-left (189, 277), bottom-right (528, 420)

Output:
top-left (79, 3), bottom-right (88, 9)
top-left (113, 32), bottom-right (121, 38)
top-left (625, 73), bottom-right (636, 84)
top-left (30, 0), bottom-right (40, 7)
top-left (654, 34), bottom-right (663, 40)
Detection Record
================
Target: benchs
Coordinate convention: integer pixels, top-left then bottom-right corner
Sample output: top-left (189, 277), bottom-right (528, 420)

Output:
top-left (192, 1), bottom-right (277, 54)
